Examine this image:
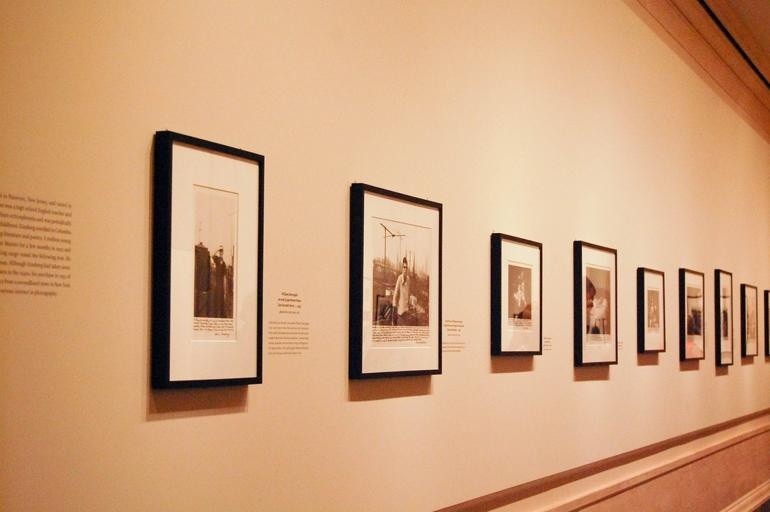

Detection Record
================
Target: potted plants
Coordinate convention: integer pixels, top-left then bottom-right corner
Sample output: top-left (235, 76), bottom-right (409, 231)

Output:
top-left (572, 240), bottom-right (618, 367)
top-left (679, 268), bottom-right (705, 361)
top-left (764, 290), bottom-right (770, 356)
top-left (637, 267), bottom-right (666, 353)
top-left (740, 283), bottom-right (758, 356)
top-left (491, 232), bottom-right (542, 355)
top-left (714, 269), bottom-right (734, 366)
top-left (348, 181), bottom-right (443, 377)
top-left (149, 130), bottom-right (265, 391)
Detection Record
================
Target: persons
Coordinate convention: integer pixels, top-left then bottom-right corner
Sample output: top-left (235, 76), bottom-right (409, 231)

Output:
top-left (392, 257), bottom-right (411, 326)
top-left (586, 276), bottom-right (601, 334)
top-left (212, 243), bottom-right (228, 317)
